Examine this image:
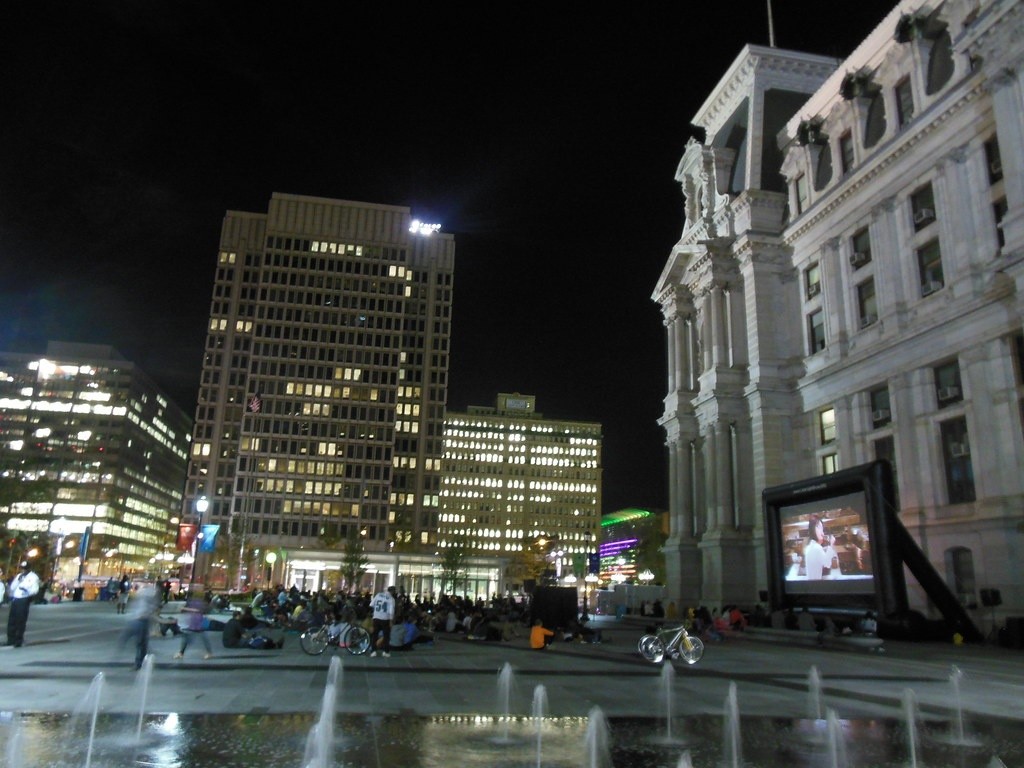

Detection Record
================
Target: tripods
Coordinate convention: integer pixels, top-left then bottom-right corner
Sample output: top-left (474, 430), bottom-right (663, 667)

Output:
top-left (983, 606), bottom-right (1000, 647)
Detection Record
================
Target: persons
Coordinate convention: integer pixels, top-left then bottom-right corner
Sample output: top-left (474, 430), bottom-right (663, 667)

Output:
top-left (770, 605), bottom-right (877, 645)
top-left (0, 560), bottom-right (40, 648)
top-left (530, 619), bottom-right (602, 650)
top-left (110, 578), bottom-right (163, 672)
top-left (203, 585), bottom-right (531, 657)
top-left (639, 598), bottom-right (745, 643)
top-left (174, 583), bottom-right (213, 660)
top-left (108, 574), bottom-right (190, 613)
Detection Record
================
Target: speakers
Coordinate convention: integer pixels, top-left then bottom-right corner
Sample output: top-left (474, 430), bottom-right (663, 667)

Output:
top-left (759, 591), bottom-right (769, 602)
top-left (979, 588), bottom-right (1003, 607)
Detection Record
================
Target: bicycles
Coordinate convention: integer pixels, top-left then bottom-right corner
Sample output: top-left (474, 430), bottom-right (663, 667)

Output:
top-left (638, 615), bottom-right (705, 665)
top-left (300, 607), bottom-right (372, 655)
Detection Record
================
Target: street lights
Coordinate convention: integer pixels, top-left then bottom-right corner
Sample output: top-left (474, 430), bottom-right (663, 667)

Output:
top-left (186, 494), bottom-right (209, 602)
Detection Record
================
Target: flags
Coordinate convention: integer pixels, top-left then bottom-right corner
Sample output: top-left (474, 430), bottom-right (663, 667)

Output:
top-left (176, 523), bottom-right (197, 551)
top-left (199, 525), bottom-right (220, 552)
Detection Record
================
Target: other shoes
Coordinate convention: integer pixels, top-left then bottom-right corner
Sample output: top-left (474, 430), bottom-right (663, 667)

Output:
top-left (871, 647), bottom-right (875, 650)
top-left (174, 654), bottom-right (183, 659)
top-left (371, 651), bottom-right (377, 657)
top-left (382, 651), bottom-right (391, 657)
top-left (204, 653), bottom-right (211, 659)
top-left (277, 637), bottom-right (284, 648)
top-left (879, 647), bottom-right (885, 651)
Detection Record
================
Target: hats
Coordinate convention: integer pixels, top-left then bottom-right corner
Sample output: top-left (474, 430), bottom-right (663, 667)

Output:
top-left (19, 560), bottom-right (31, 568)
top-left (580, 616), bottom-right (590, 621)
